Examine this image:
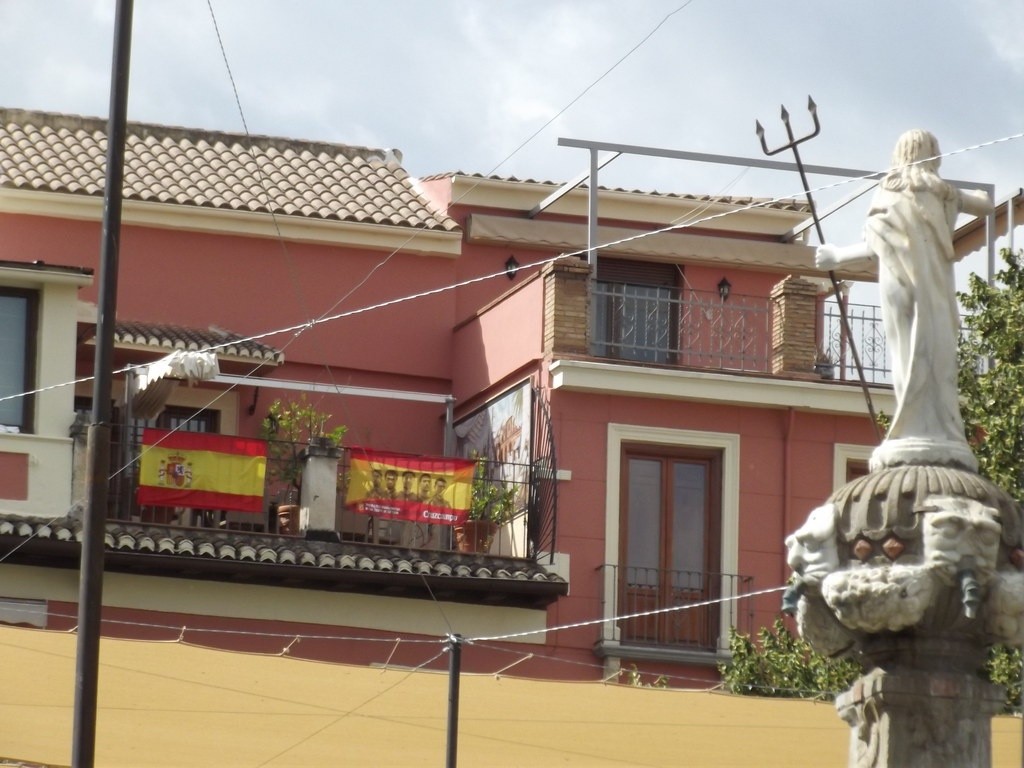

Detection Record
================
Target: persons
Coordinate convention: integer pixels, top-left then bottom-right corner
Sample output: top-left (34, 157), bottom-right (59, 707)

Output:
top-left (365, 468), bottom-right (453, 509)
top-left (816, 129), bottom-right (996, 473)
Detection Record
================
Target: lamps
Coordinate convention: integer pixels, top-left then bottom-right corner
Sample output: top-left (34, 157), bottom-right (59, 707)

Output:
top-left (505, 254), bottom-right (519, 280)
top-left (717, 276), bottom-right (731, 301)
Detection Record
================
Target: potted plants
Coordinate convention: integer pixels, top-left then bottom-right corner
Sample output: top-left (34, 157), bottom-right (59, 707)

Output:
top-left (454, 450), bottom-right (520, 553)
top-left (260, 393), bottom-right (350, 535)
top-left (815, 342), bottom-right (835, 379)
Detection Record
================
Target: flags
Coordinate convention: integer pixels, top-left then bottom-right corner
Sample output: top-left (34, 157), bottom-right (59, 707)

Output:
top-left (136, 428), bottom-right (268, 512)
top-left (344, 447), bottom-right (477, 527)
